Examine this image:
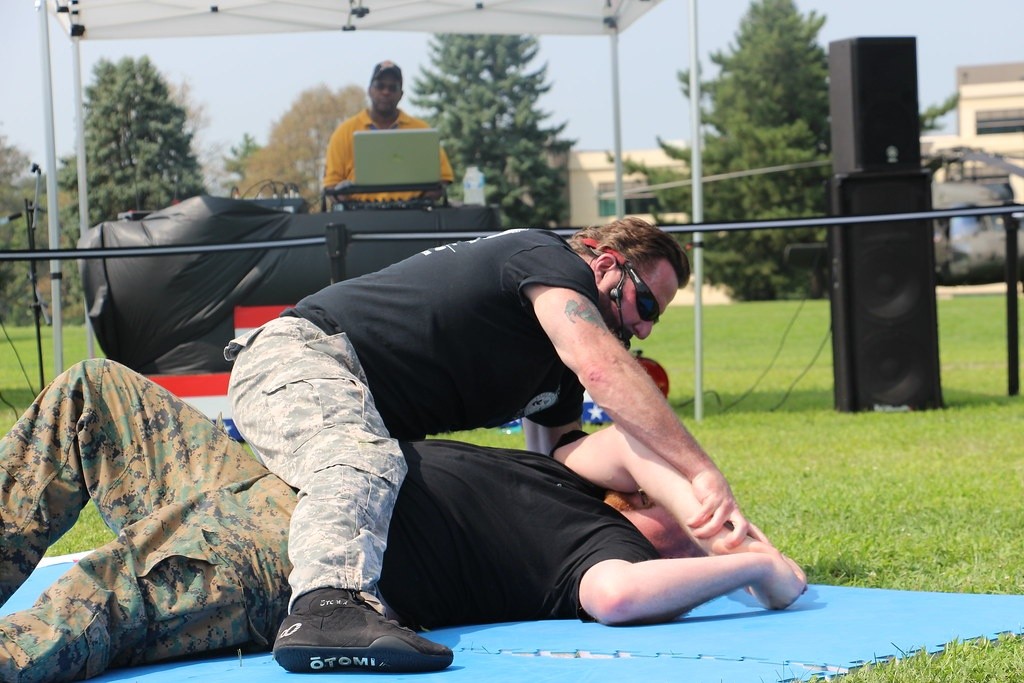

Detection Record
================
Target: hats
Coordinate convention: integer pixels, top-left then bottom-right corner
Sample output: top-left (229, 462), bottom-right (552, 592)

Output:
top-left (372, 60), bottom-right (403, 82)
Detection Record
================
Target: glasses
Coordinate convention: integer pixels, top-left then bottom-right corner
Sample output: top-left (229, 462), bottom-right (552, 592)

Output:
top-left (580, 235), bottom-right (661, 324)
top-left (371, 82), bottom-right (401, 92)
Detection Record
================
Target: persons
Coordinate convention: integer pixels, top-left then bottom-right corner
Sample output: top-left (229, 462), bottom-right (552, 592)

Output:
top-left (0, 219), bottom-right (808, 683)
top-left (324, 60), bottom-right (454, 213)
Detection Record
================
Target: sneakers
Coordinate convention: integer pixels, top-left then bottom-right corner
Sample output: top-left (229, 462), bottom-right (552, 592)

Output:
top-left (273, 584), bottom-right (453, 674)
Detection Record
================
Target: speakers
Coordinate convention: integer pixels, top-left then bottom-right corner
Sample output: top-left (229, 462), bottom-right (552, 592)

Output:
top-left (828, 36), bottom-right (922, 176)
top-left (826, 171), bottom-right (943, 414)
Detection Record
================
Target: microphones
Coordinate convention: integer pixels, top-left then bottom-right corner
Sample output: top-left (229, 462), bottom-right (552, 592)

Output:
top-left (609, 289), bottom-right (631, 352)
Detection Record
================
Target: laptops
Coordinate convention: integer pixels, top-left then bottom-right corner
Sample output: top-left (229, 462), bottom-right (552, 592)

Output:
top-left (352, 130), bottom-right (441, 187)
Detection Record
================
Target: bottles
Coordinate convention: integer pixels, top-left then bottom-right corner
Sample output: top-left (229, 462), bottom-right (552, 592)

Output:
top-left (463, 167), bottom-right (485, 206)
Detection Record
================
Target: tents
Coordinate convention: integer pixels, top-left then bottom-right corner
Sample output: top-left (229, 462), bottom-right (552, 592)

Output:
top-left (37, 0), bottom-right (705, 424)
